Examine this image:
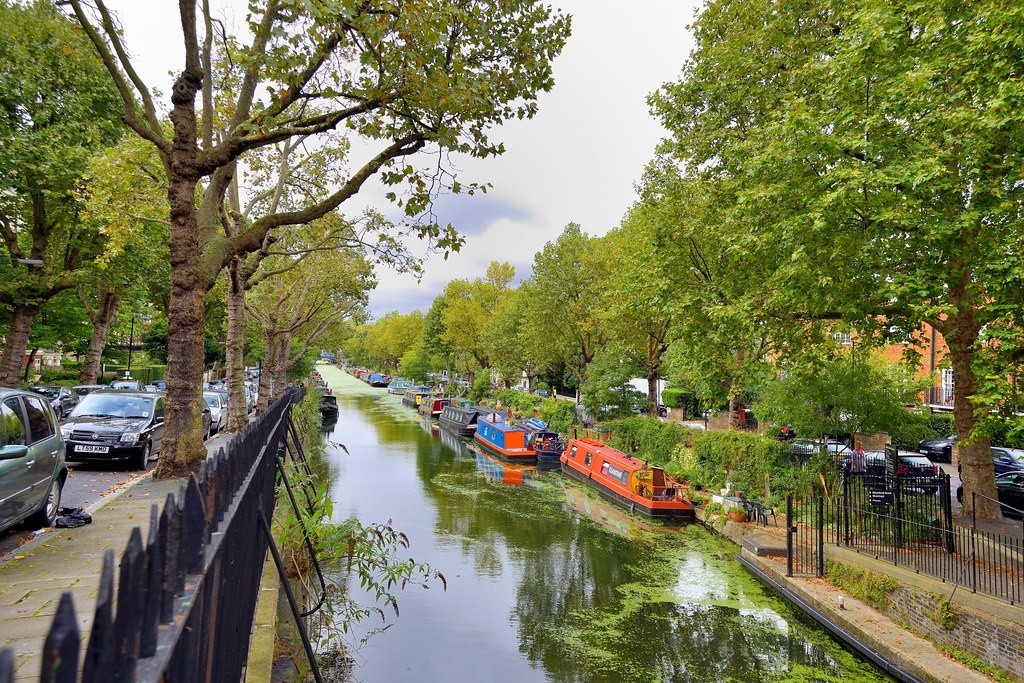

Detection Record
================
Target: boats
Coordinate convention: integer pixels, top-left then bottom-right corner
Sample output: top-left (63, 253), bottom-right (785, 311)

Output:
top-left (310, 366), bottom-right (334, 395)
top-left (419, 397), bottom-right (450, 421)
top-left (466, 443), bottom-right (539, 488)
top-left (560, 428), bottom-right (696, 526)
top-left (401, 389), bottom-right (427, 408)
top-left (472, 407), bottom-right (553, 466)
top-left (437, 404), bottom-right (483, 439)
top-left (558, 479), bottom-right (682, 542)
top-left (318, 415), bottom-right (337, 432)
top-left (389, 379), bottom-right (413, 396)
top-left (318, 394), bottom-right (340, 418)
top-left (337, 361), bottom-right (389, 387)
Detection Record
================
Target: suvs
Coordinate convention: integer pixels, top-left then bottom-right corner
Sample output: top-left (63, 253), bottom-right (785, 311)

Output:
top-left (787, 439), bottom-right (854, 477)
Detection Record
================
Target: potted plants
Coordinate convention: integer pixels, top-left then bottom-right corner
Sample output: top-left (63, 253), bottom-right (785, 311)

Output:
top-left (687, 493), bottom-right (709, 505)
top-left (725, 506), bottom-right (749, 522)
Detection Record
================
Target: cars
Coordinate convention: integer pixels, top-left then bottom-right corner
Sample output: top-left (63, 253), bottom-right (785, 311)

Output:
top-left (843, 451), bottom-right (939, 495)
top-left (512, 386), bottom-right (549, 399)
top-left (956, 448), bottom-right (1024, 477)
top-left (0, 388), bottom-right (68, 534)
top-left (73, 365), bottom-right (261, 440)
top-left (430, 375), bottom-right (448, 385)
top-left (60, 389), bottom-right (171, 470)
top-left (955, 471), bottom-right (1024, 513)
top-left (23, 386), bottom-right (81, 421)
top-left (455, 378), bottom-right (472, 388)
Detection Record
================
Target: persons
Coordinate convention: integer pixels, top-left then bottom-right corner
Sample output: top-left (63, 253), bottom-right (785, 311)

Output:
top-left (285, 381), bottom-right (294, 396)
top-left (328, 389), bottom-right (332, 395)
top-left (850, 441), bottom-right (868, 486)
top-left (553, 387), bottom-right (557, 401)
top-left (781, 424), bottom-right (792, 440)
top-left (547, 387), bottom-right (552, 396)
top-left (300, 383), bottom-right (304, 387)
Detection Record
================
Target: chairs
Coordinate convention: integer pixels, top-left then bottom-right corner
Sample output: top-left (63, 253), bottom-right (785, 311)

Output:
top-left (746, 501), bottom-right (779, 528)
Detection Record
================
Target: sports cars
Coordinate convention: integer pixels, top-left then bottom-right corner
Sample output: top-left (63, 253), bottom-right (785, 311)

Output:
top-left (920, 433), bottom-right (959, 462)
